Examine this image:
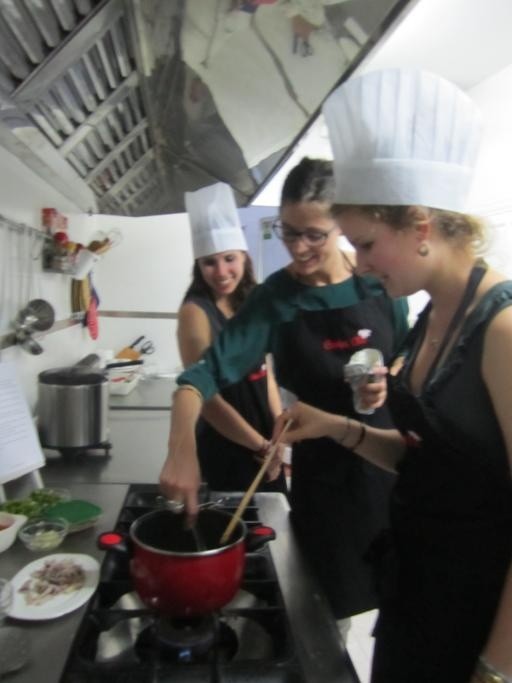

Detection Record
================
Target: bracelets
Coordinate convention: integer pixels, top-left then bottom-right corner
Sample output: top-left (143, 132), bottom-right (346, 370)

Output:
top-left (343, 422), bottom-right (365, 453)
top-left (258, 439), bottom-right (270, 458)
top-left (474, 656), bottom-right (511, 683)
top-left (337, 417), bottom-right (351, 444)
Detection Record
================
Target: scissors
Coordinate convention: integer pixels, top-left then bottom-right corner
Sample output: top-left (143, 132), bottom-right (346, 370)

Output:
top-left (139, 340), bottom-right (155, 355)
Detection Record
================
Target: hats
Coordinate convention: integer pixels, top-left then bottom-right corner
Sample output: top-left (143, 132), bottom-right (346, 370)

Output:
top-left (183, 180), bottom-right (249, 260)
top-left (323, 68), bottom-right (481, 212)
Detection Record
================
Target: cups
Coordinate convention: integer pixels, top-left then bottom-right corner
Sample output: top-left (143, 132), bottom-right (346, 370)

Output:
top-left (341, 346), bottom-right (386, 416)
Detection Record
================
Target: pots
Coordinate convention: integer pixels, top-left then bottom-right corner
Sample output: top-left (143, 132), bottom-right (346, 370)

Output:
top-left (34, 368), bottom-right (109, 447)
top-left (96, 508), bottom-right (276, 623)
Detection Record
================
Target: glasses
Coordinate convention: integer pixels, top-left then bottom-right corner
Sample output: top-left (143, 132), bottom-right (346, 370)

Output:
top-left (270, 217), bottom-right (337, 246)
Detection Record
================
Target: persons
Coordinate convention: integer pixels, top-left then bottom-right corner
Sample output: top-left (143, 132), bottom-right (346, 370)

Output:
top-left (177, 249), bottom-right (287, 492)
top-left (271, 203), bottom-right (512, 683)
top-left (159, 157), bottom-right (408, 643)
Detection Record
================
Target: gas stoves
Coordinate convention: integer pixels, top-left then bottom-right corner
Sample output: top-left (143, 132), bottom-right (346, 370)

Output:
top-left (55, 480), bottom-right (306, 682)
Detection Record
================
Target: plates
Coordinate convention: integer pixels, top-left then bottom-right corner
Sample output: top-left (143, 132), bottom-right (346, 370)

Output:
top-left (1, 553), bottom-right (102, 623)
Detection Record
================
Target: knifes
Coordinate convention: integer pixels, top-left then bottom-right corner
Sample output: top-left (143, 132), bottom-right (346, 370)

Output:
top-left (127, 336), bottom-right (146, 350)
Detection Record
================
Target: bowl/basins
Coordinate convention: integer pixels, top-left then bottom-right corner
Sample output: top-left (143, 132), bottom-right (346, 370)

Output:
top-left (0, 510), bottom-right (26, 552)
top-left (17, 518), bottom-right (67, 554)
